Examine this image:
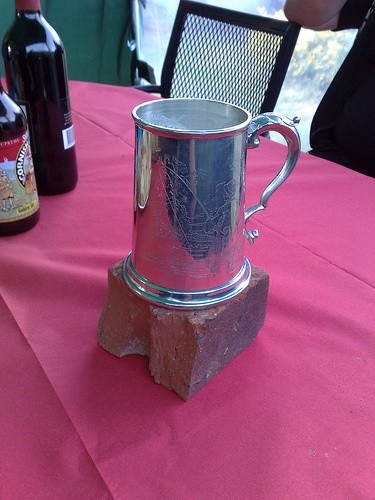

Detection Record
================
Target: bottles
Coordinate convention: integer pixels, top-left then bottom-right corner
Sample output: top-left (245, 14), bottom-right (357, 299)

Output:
top-left (0, 79), bottom-right (40, 237)
top-left (3, 0), bottom-right (78, 195)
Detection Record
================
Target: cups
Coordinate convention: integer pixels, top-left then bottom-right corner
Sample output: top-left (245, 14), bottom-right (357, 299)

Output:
top-left (121, 98), bottom-right (300, 310)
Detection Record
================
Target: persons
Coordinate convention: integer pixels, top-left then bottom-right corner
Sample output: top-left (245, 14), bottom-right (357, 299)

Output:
top-left (284, 0), bottom-right (375, 178)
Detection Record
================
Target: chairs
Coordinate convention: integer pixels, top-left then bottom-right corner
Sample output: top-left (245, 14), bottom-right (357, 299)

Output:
top-left (132, 0), bottom-right (301, 138)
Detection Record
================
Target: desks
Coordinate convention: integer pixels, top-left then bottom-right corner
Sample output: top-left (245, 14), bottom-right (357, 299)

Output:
top-left (0, 74), bottom-right (375, 500)
